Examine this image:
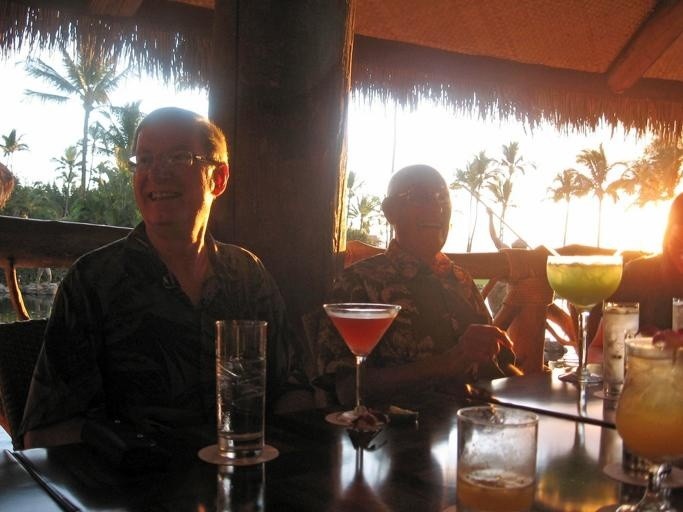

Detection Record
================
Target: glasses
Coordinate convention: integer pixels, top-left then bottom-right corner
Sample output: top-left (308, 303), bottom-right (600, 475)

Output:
top-left (394, 188), bottom-right (448, 206)
top-left (127, 151), bottom-right (213, 172)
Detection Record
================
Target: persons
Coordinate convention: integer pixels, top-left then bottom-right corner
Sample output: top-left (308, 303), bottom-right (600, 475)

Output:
top-left (317, 164), bottom-right (525, 410)
top-left (586, 193), bottom-right (683, 366)
top-left (12, 106), bottom-right (313, 449)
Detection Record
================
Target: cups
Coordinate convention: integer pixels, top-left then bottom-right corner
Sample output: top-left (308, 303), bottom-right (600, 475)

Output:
top-left (456, 406), bottom-right (539, 512)
top-left (216, 318), bottom-right (267, 459)
top-left (603, 302), bottom-right (639, 402)
top-left (216, 462), bottom-right (265, 512)
top-left (672, 297), bottom-right (683, 331)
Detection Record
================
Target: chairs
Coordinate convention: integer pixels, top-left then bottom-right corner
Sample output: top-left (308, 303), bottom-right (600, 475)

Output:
top-left (1, 319), bottom-right (50, 451)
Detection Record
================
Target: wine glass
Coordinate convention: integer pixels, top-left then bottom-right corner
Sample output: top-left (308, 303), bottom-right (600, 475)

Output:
top-left (614, 337), bottom-right (682, 512)
top-left (332, 428), bottom-right (394, 511)
top-left (546, 254), bottom-right (623, 385)
top-left (322, 302), bottom-right (401, 426)
top-left (538, 387), bottom-right (618, 511)
